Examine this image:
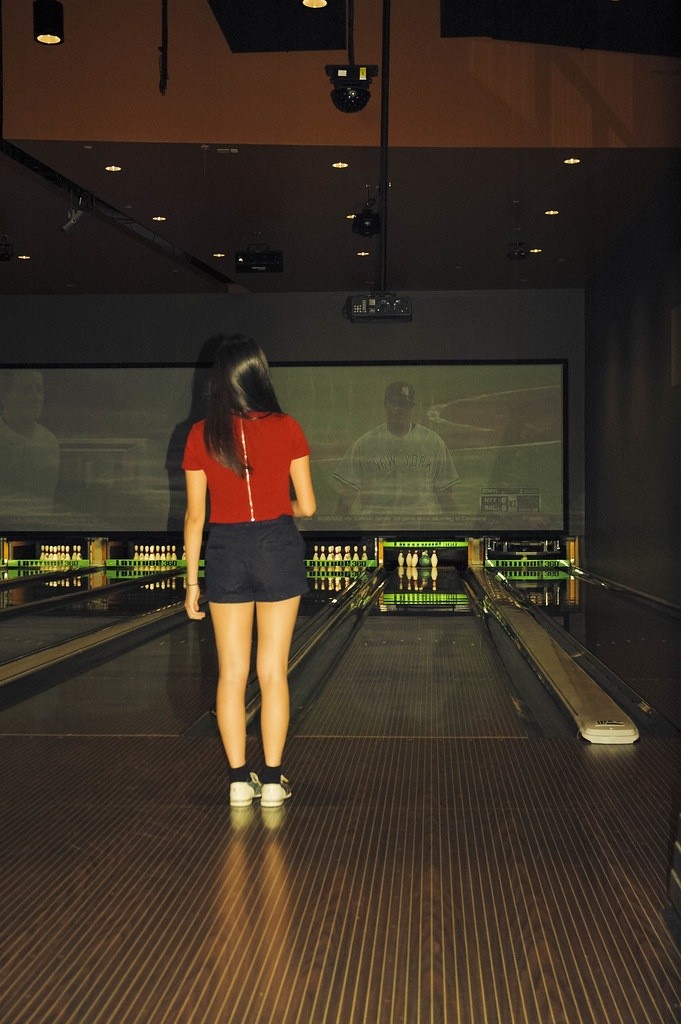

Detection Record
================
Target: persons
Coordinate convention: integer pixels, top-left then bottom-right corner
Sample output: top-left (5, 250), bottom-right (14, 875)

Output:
top-left (2, 369), bottom-right (59, 529)
top-left (182, 334), bottom-right (316, 810)
top-left (333, 381), bottom-right (460, 516)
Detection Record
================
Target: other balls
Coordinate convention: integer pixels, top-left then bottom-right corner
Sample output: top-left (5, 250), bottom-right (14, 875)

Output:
top-left (419, 555), bottom-right (431, 567)
top-left (419, 570), bottom-right (430, 577)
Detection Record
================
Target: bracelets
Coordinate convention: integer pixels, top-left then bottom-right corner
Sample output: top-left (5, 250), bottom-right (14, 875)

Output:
top-left (183, 582), bottom-right (200, 588)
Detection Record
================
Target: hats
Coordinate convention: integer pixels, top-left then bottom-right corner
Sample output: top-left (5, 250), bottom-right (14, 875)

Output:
top-left (384, 380), bottom-right (418, 407)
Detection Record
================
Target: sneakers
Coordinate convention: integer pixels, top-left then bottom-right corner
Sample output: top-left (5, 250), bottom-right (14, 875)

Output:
top-left (229, 796), bottom-right (260, 829)
top-left (230, 772), bottom-right (265, 806)
top-left (260, 773), bottom-right (290, 808)
top-left (260, 795), bottom-right (291, 830)
